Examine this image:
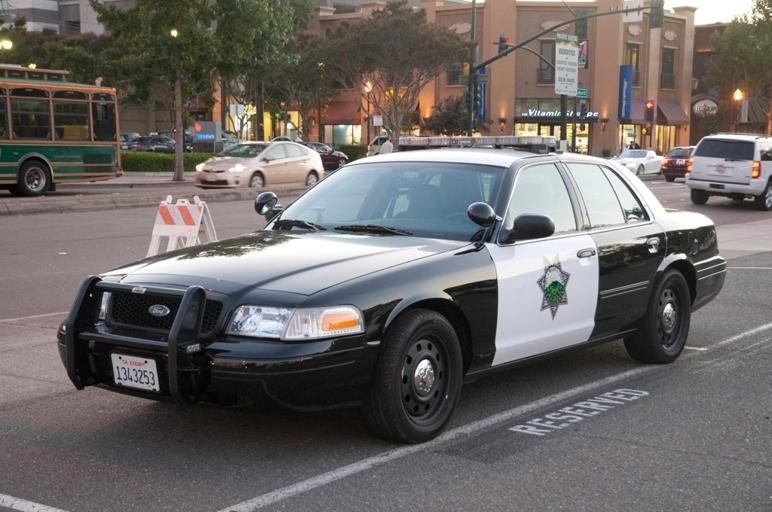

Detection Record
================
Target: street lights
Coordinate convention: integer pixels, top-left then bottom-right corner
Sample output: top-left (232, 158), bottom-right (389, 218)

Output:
top-left (733, 88), bottom-right (743, 133)
top-left (316, 57), bottom-right (327, 140)
top-left (363, 79), bottom-right (373, 145)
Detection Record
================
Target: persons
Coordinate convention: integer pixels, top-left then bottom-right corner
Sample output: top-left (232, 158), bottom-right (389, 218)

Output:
top-left (380, 135), bottom-right (394, 154)
top-left (629, 141), bottom-right (640, 149)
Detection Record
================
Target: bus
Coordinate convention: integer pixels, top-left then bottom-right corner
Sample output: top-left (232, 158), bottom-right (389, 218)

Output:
top-left (0, 67), bottom-right (122, 196)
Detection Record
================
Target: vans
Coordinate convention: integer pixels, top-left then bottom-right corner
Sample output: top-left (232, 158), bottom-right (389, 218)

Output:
top-left (367, 135), bottom-right (390, 155)
top-left (684, 133), bottom-right (771, 211)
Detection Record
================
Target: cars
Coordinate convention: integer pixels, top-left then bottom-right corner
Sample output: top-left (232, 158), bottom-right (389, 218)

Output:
top-left (57, 137), bottom-right (728, 444)
top-left (608, 146), bottom-right (697, 181)
top-left (195, 141), bottom-right (324, 190)
top-left (120, 130), bottom-right (192, 153)
top-left (297, 142), bottom-right (349, 168)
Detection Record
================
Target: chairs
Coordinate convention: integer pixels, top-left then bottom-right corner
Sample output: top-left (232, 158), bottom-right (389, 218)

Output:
top-left (393, 185), bottom-right (445, 223)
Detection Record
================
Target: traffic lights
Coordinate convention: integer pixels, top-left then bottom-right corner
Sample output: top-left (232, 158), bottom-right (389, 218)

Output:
top-left (645, 100), bottom-right (655, 120)
top-left (497, 33), bottom-right (508, 57)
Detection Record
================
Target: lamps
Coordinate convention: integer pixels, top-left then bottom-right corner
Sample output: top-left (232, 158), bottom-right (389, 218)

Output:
top-left (600, 118), bottom-right (609, 132)
top-left (641, 127), bottom-right (651, 136)
top-left (498, 117), bottom-right (506, 134)
